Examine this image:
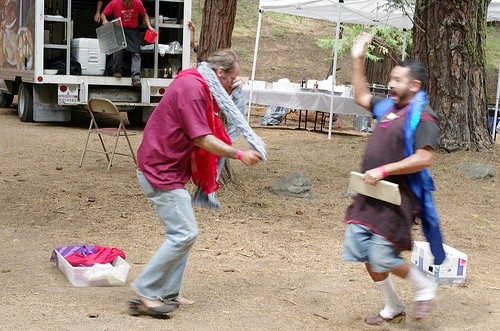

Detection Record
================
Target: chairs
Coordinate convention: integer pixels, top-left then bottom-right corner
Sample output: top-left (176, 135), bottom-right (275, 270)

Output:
top-left (79, 97), bottom-right (137, 171)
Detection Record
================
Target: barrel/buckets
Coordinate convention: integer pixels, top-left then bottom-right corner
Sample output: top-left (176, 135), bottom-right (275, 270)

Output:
top-left (143, 29), bottom-right (157, 44)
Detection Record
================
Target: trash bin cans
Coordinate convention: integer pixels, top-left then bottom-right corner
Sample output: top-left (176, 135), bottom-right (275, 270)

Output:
top-left (488, 104), bottom-right (500, 140)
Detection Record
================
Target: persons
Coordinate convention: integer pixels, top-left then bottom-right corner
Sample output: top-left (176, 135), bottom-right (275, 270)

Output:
top-left (129, 48), bottom-right (262, 314)
top-left (340, 31), bottom-right (442, 325)
top-left (94, 0), bottom-right (155, 86)
top-left (187, 21), bottom-right (243, 90)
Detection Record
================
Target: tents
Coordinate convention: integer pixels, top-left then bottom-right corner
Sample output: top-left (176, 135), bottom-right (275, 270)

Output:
top-left (247, 0), bottom-right (500, 141)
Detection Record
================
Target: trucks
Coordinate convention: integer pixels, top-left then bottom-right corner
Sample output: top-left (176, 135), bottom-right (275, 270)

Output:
top-left (0, 0), bottom-right (194, 126)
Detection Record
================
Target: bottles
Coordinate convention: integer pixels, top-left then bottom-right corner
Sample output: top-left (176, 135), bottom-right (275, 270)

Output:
top-left (159, 15), bottom-right (163, 24)
top-left (168, 68), bottom-right (172, 79)
top-left (163, 68), bottom-right (168, 78)
top-left (302, 79), bottom-right (306, 89)
top-left (314, 82), bottom-right (318, 93)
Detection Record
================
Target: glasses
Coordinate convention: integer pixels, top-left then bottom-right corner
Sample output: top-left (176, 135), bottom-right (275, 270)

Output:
top-left (223, 72), bottom-right (237, 85)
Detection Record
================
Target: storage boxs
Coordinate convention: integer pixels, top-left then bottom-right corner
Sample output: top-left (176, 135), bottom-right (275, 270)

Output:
top-left (55, 248), bottom-right (130, 288)
top-left (96, 16), bottom-right (127, 55)
top-left (70, 38), bottom-right (106, 76)
top-left (411, 241), bottom-right (468, 285)
top-left (141, 68), bottom-right (169, 79)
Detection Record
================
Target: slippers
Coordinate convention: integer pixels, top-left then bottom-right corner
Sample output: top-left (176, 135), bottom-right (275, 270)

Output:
top-left (365, 311), bottom-right (406, 325)
top-left (415, 284), bottom-right (437, 320)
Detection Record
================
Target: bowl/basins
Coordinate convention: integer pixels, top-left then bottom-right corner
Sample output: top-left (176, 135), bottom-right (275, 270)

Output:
top-left (163, 18), bottom-right (177, 24)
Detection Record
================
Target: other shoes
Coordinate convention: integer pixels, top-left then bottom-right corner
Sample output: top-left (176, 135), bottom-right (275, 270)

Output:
top-left (132, 80), bottom-right (141, 85)
top-left (127, 299), bottom-right (176, 313)
top-left (114, 72), bottom-right (121, 77)
top-left (162, 297), bottom-right (181, 306)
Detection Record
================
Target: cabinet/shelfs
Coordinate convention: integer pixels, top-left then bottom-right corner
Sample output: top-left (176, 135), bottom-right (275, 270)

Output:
top-left (140, 23), bottom-right (182, 55)
top-left (44, 16), bottom-right (69, 49)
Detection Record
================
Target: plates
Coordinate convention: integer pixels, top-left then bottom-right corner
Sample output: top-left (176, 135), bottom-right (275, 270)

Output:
top-left (43, 69), bottom-right (58, 75)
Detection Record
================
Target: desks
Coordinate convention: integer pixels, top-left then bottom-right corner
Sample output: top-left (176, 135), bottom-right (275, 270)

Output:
top-left (245, 88), bottom-right (376, 133)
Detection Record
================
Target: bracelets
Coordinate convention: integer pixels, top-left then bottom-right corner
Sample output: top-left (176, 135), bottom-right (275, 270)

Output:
top-left (236, 150), bottom-right (243, 160)
top-left (380, 165), bottom-right (388, 178)
